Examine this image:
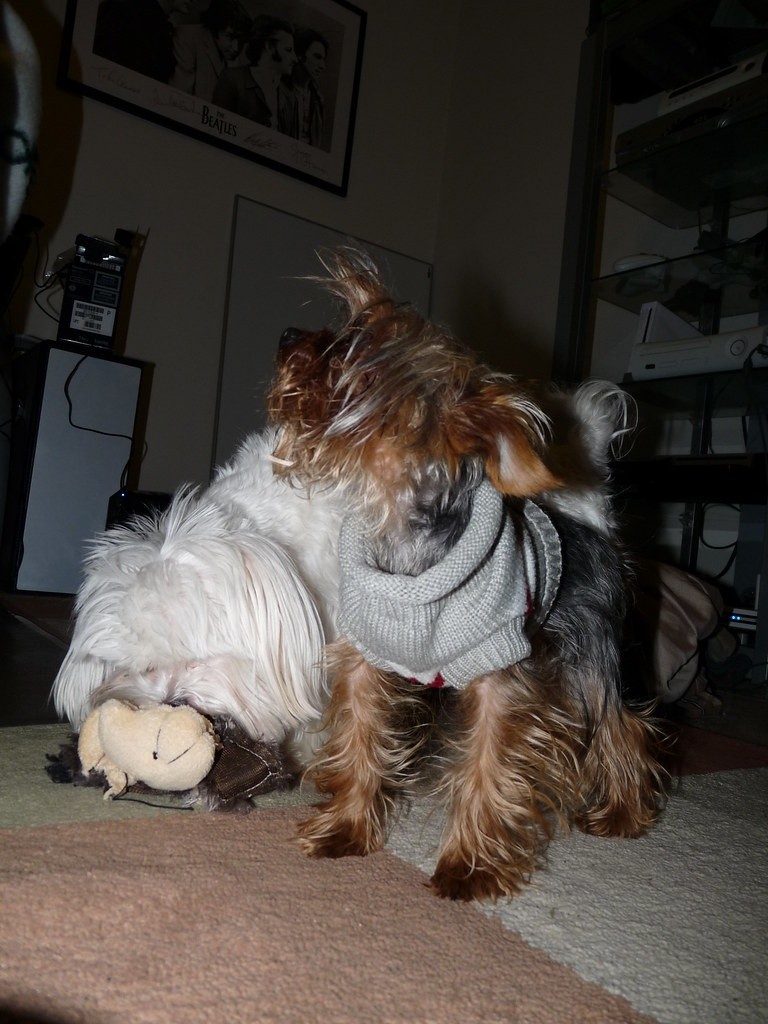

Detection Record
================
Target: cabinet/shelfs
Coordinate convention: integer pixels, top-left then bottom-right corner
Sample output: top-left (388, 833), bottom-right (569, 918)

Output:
top-left (0, 339), bottom-right (156, 597)
top-left (551, 0), bottom-right (767, 747)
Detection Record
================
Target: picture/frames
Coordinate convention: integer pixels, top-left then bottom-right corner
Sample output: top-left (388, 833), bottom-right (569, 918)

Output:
top-left (58, 0), bottom-right (368, 199)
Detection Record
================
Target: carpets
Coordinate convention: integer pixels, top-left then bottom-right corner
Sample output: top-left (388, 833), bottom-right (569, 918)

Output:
top-left (0, 723), bottom-right (768, 1023)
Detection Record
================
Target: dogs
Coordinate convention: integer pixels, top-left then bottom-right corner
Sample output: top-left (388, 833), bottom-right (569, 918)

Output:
top-left (46, 240), bottom-right (683, 901)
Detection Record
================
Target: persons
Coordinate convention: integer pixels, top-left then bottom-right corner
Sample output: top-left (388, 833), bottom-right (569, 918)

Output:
top-left (87, 0), bottom-right (331, 185)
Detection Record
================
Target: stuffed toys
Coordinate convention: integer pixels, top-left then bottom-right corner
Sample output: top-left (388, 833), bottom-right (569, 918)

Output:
top-left (78, 699), bottom-right (225, 800)
